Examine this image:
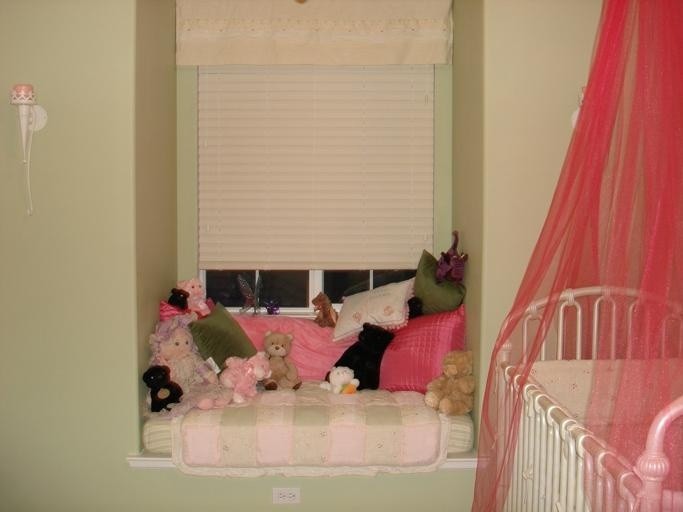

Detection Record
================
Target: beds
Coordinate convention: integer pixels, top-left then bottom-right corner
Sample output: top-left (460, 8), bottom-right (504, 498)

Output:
top-left (494, 286), bottom-right (683, 512)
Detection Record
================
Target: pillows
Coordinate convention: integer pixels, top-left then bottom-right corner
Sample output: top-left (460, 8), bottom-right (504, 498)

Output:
top-left (187, 302), bottom-right (257, 374)
top-left (377, 304), bottom-right (466, 395)
top-left (415, 249), bottom-right (466, 313)
top-left (521, 358), bottom-right (683, 427)
top-left (331, 277), bottom-right (415, 343)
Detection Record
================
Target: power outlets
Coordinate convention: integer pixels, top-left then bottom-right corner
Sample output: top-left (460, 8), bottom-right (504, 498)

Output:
top-left (272, 487), bottom-right (300, 503)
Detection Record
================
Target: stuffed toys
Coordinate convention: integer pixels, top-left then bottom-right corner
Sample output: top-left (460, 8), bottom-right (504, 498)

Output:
top-left (423, 349), bottom-right (476, 416)
top-left (141, 313), bottom-right (234, 417)
top-left (176, 276), bottom-right (210, 317)
top-left (141, 364), bottom-right (183, 412)
top-left (167, 287), bottom-right (189, 310)
top-left (217, 350), bottom-right (273, 404)
top-left (323, 321), bottom-right (395, 390)
top-left (434, 229), bottom-right (469, 288)
top-left (317, 366), bottom-right (361, 395)
top-left (407, 295), bottom-right (425, 320)
top-left (261, 329), bottom-right (303, 390)
top-left (310, 291), bottom-right (338, 328)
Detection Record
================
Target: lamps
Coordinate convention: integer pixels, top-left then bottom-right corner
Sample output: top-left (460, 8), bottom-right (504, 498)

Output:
top-left (9, 84), bottom-right (48, 164)
